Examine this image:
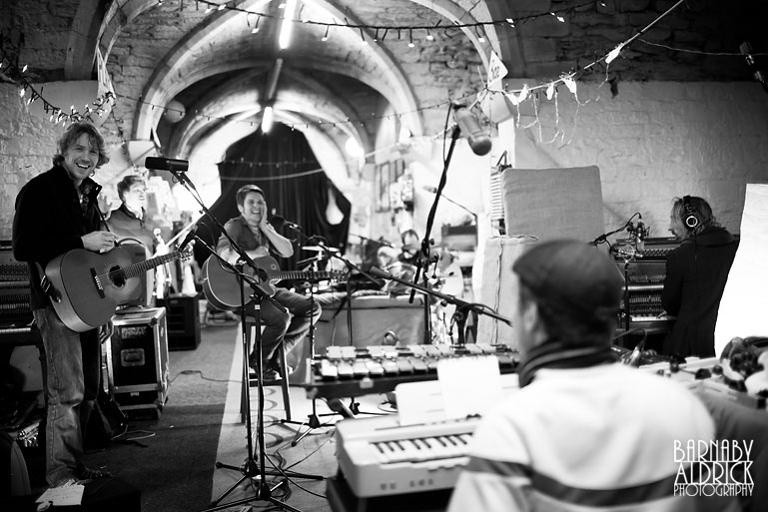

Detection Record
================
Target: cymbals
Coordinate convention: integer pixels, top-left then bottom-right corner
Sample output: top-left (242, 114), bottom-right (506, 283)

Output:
top-left (300, 244), bottom-right (339, 252)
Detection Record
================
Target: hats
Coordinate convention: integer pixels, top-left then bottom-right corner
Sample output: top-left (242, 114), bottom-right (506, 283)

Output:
top-left (511, 242), bottom-right (626, 315)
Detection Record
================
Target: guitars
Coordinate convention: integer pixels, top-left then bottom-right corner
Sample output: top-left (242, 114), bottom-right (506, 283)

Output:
top-left (45, 244), bottom-right (191, 333)
top-left (200, 252), bottom-right (351, 312)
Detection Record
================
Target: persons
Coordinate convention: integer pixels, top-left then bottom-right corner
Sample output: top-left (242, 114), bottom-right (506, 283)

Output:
top-left (9, 120), bottom-right (119, 488)
top-left (442, 238), bottom-right (744, 511)
top-left (216, 184), bottom-right (323, 383)
top-left (97, 174), bottom-right (164, 309)
top-left (654, 194), bottom-right (740, 359)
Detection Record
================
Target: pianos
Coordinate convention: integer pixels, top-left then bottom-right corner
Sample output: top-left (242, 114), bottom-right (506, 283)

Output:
top-left (335, 396), bottom-right (507, 498)
top-left (617, 274), bottom-right (677, 351)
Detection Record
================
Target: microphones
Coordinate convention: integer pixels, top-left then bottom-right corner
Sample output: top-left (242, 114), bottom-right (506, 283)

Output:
top-left (452, 107), bottom-right (492, 158)
top-left (143, 156), bottom-right (190, 172)
top-left (179, 225), bottom-right (200, 251)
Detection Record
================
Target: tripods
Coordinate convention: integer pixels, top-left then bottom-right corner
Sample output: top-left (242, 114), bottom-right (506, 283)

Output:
top-left (203, 187), bottom-right (388, 512)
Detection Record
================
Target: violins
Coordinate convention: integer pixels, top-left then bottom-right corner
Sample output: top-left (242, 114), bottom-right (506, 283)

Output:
top-left (154, 228), bottom-right (174, 300)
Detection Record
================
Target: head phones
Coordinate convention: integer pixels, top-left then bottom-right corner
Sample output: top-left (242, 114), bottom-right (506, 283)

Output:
top-left (682, 195), bottom-right (699, 229)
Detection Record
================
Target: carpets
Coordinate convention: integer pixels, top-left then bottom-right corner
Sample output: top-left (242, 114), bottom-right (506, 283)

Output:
top-left (57, 302), bottom-right (239, 510)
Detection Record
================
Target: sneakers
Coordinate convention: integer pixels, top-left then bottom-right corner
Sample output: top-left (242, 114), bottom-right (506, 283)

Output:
top-left (79, 468), bottom-right (112, 480)
top-left (249, 354), bottom-right (280, 382)
top-left (55, 478), bottom-right (104, 499)
top-left (272, 358), bottom-right (293, 375)
